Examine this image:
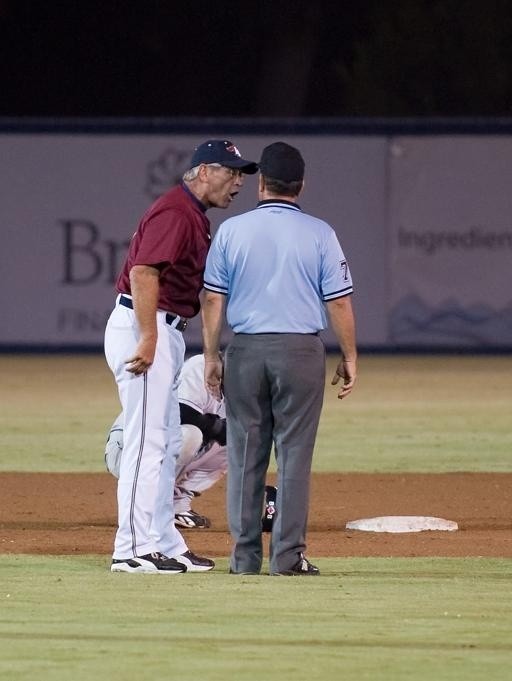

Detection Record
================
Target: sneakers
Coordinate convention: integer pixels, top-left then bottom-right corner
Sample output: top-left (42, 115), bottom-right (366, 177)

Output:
top-left (172, 550), bottom-right (215, 573)
top-left (174, 510), bottom-right (211, 528)
top-left (110, 552), bottom-right (187, 574)
top-left (273, 551), bottom-right (319, 575)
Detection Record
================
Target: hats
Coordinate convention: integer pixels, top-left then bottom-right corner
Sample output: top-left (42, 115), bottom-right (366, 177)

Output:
top-left (257, 142), bottom-right (305, 182)
top-left (188, 139), bottom-right (259, 174)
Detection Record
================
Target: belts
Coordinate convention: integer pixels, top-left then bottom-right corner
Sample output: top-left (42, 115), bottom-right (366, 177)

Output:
top-left (119, 294), bottom-right (188, 332)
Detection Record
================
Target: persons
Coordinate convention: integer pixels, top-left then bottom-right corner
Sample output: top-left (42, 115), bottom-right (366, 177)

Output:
top-left (202, 142), bottom-right (357, 575)
top-left (104, 139), bottom-right (259, 575)
top-left (103, 353), bottom-right (229, 529)
